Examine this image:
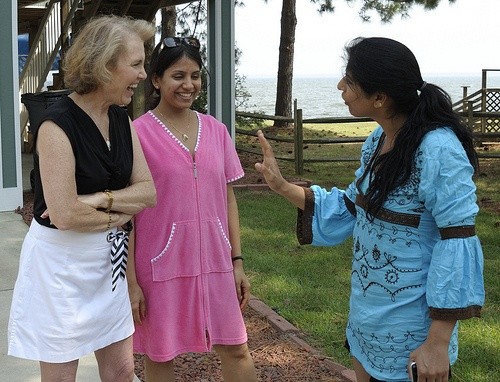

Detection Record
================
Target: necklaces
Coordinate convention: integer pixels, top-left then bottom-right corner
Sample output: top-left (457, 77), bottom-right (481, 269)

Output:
top-left (69, 95), bottom-right (109, 141)
top-left (157, 107), bottom-right (191, 143)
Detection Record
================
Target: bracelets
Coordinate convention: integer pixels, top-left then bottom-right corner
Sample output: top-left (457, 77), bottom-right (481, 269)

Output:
top-left (103, 189), bottom-right (114, 213)
top-left (102, 212), bottom-right (112, 232)
top-left (232, 256), bottom-right (244, 261)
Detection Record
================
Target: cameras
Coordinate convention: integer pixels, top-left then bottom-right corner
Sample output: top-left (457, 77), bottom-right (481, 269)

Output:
top-left (407, 361), bottom-right (451, 382)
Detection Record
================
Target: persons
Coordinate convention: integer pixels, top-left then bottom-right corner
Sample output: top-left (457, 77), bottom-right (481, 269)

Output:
top-left (255, 37), bottom-right (484, 382)
top-left (126, 36), bottom-right (258, 382)
top-left (8, 17), bottom-right (157, 382)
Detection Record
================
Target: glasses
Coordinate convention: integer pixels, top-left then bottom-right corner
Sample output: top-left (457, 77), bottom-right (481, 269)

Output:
top-left (157, 36), bottom-right (200, 59)
